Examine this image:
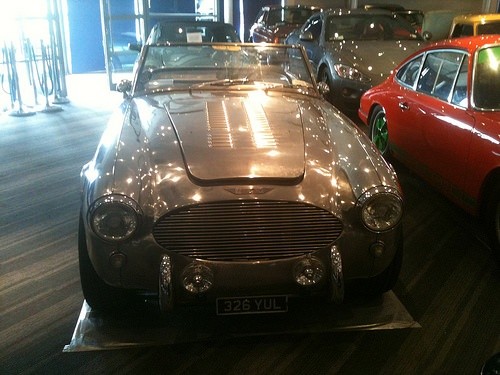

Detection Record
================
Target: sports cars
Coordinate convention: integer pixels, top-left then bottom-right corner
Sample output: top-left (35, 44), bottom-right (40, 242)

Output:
top-left (73, 41), bottom-right (408, 322)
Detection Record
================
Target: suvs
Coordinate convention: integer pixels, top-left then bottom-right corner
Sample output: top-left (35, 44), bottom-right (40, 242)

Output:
top-left (128, 20), bottom-right (239, 62)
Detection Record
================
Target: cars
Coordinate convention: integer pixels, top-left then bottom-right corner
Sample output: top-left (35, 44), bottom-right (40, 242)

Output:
top-left (448, 13), bottom-right (500, 45)
top-left (358, 31), bottom-right (500, 227)
top-left (249, 4), bottom-right (318, 44)
top-left (285, 8), bottom-right (430, 114)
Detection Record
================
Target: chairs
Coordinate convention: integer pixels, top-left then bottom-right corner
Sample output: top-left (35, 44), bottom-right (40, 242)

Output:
top-left (363, 18), bottom-right (386, 40)
